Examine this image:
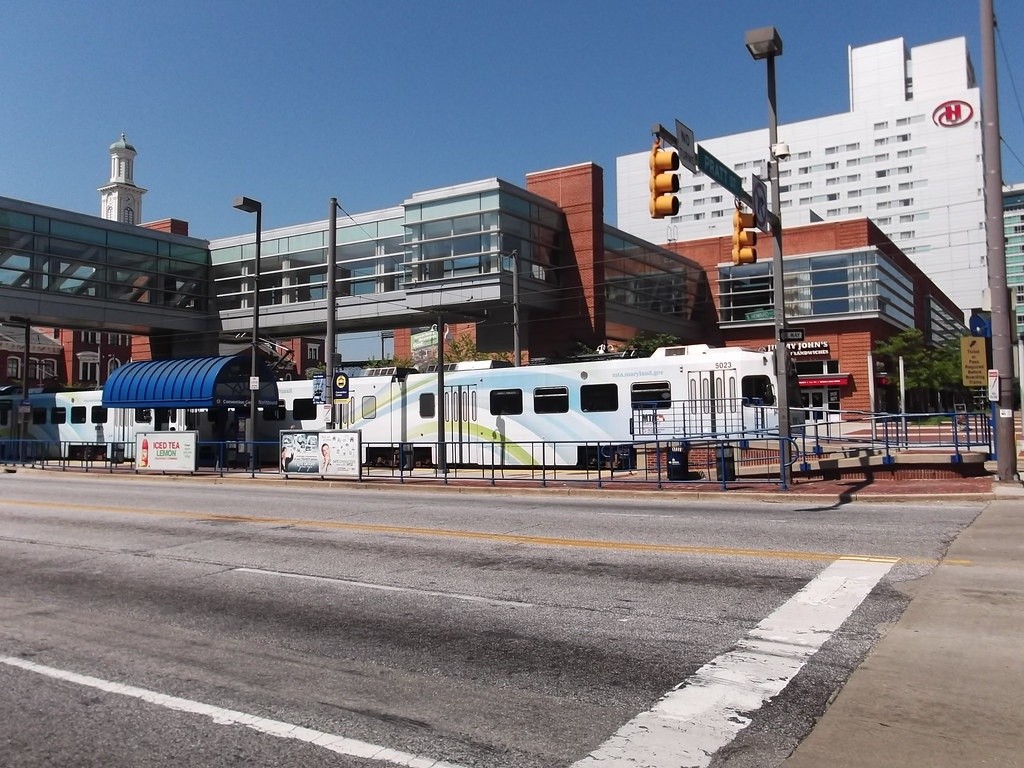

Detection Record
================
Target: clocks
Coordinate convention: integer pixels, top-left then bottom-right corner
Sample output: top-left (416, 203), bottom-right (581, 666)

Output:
top-left (124, 193), bottom-right (135, 205)
top-left (106, 192), bottom-right (114, 206)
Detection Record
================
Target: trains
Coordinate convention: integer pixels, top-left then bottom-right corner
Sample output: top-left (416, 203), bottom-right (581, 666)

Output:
top-left (0, 342), bottom-right (804, 466)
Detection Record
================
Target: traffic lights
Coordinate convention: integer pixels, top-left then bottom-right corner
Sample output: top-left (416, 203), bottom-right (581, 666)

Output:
top-left (649, 145), bottom-right (679, 219)
top-left (733, 209), bottom-right (758, 267)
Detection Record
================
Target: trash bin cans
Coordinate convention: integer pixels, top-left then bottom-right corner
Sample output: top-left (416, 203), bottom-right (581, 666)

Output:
top-left (666, 438), bottom-right (689, 481)
top-left (716, 447), bottom-right (735, 480)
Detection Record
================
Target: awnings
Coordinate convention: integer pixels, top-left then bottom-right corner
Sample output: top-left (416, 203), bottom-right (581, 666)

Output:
top-left (876, 372), bottom-right (897, 385)
top-left (797, 372), bottom-right (851, 386)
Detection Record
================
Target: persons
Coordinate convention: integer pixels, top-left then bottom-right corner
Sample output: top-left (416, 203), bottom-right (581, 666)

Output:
top-left (319, 443), bottom-right (339, 474)
top-left (281, 447), bottom-right (289, 470)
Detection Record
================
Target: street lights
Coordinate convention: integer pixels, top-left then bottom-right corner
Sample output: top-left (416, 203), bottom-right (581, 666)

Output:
top-left (233, 196), bottom-right (264, 471)
top-left (744, 27), bottom-right (794, 485)
top-left (9, 315), bottom-right (33, 462)
